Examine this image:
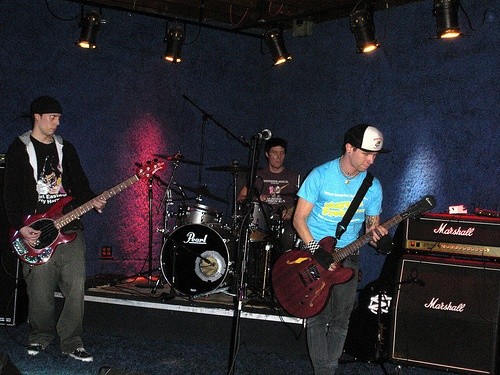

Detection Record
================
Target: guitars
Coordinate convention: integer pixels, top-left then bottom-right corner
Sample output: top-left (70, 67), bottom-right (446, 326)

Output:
top-left (8, 158), bottom-right (166, 265)
top-left (271, 195), bottom-right (436, 319)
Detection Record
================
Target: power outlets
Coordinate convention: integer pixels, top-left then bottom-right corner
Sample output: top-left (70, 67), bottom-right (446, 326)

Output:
top-left (100, 246), bottom-right (112, 258)
top-left (484, 9), bottom-right (495, 23)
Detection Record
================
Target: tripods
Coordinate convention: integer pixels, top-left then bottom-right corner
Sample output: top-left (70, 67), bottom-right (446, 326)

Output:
top-left (149, 165), bottom-right (279, 302)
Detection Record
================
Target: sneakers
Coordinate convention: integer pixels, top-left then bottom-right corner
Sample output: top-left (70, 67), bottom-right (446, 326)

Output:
top-left (63, 347), bottom-right (93, 362)
top-left (26, 343), bottom-right (40, 356)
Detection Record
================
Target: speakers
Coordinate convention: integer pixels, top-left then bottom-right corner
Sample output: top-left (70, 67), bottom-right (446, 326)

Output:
top-left (387, 255), bottom-right (500, 375)
top-left (0, 166), bottom-right (28, 326)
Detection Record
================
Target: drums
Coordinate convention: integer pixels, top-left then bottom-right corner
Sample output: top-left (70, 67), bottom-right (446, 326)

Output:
top-left (173, 203), bottom-right (223, 233)
top-left (159, 223), bottom-right (229, 298)
top-left (274, 220), bottom-right (298, 253)
top-left (231, 200), bottom-right (272, 243)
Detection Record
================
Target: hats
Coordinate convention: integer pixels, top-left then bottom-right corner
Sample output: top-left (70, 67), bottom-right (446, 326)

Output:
top-left (31, 95), bottom-right (62, 115)
top-left (345, 125), bottom-right (391, 154)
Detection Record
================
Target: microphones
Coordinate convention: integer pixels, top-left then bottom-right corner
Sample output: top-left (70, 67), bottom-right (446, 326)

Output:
top-left (413, 279), bottom-right (424, 287)
top-left (257, 128), bottom-right (271, 140)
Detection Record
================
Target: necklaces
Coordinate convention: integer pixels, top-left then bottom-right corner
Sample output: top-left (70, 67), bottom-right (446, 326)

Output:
top-left (341, 156), bottom-right (359, 185)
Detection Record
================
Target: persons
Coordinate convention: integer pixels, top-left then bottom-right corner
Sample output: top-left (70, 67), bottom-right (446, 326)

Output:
top-left (239, 138), bottom-right (301, 222)
top-left (291, 125), bottom-right (392, 375)
top-left (3, 95), bottom-right (108, 363)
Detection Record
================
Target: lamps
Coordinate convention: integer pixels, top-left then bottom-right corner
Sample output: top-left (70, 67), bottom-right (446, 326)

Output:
top-left (260, 20), bottom-right (293, 66)
top-left (348, 0), bottom-right (382, 54)
top-left (432, 0), bottom-right (462, 39)
top-left (163, 10), bottom-right (187, 63)
top-left (75, 2), bottom-right (104, 49)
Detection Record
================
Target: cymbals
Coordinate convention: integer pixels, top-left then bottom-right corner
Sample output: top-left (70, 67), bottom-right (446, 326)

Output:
top-left (173, 181), bottom-right (231, 205)
top-left (275, 192), bottom-right (299, 199)
top-left (154, 154), bottom-right (204, 166)
top-left (206, 165), bottom-right (250, 173)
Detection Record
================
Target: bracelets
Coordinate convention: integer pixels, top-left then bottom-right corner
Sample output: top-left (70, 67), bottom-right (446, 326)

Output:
top-left (306, 240), bottom-right (320, 255)
